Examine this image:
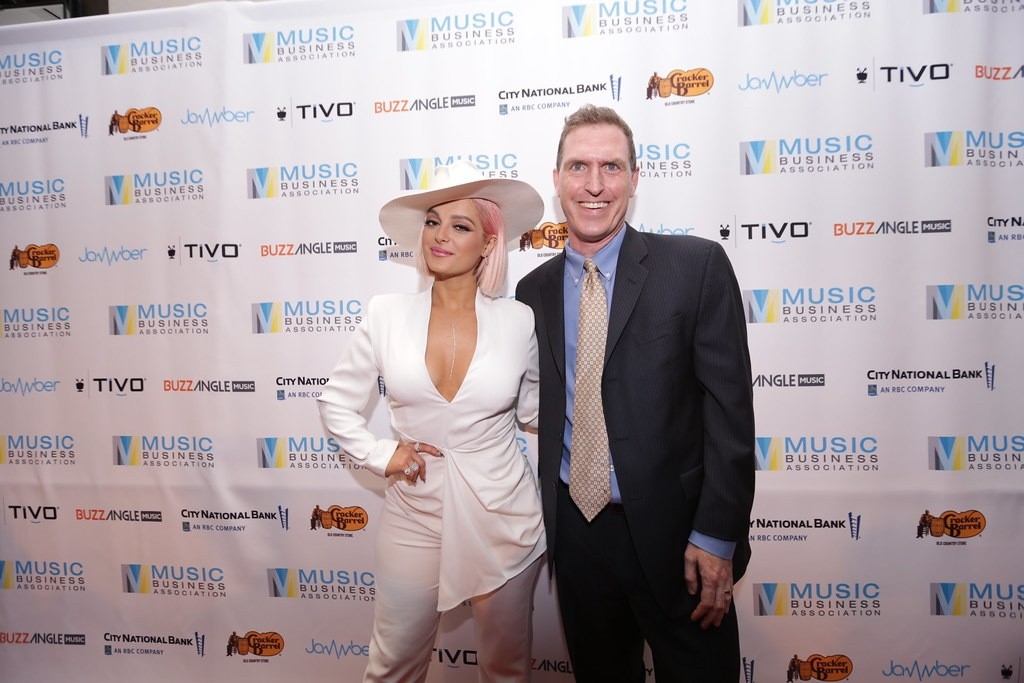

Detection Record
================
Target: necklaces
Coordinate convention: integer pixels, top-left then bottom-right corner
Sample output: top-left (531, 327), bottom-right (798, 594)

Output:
top-left (449, 324), bottom-right (456, 376)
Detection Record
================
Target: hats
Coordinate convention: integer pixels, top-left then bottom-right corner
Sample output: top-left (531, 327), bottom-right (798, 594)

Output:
top-left (379, 162), bottom-right (545, 250)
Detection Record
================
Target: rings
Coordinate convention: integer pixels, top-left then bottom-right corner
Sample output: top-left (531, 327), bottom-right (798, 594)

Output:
top-left (405, 467), bottom-right (411, 475)
top-left (414, 443), bottom-right (420, 452)
top-left (410, 461), bottom-right (419, 471)
top-left (723, 590), bottom-right (731, 596)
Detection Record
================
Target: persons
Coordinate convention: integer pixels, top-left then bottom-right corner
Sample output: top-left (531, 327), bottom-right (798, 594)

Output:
top-left (320, 160), bottom-right (539, 682)
top-left (515, 104), bottom-right (754, 682)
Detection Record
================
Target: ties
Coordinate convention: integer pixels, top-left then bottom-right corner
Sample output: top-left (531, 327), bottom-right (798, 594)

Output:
top-left (569, 257), bottom-right (612, 522)
